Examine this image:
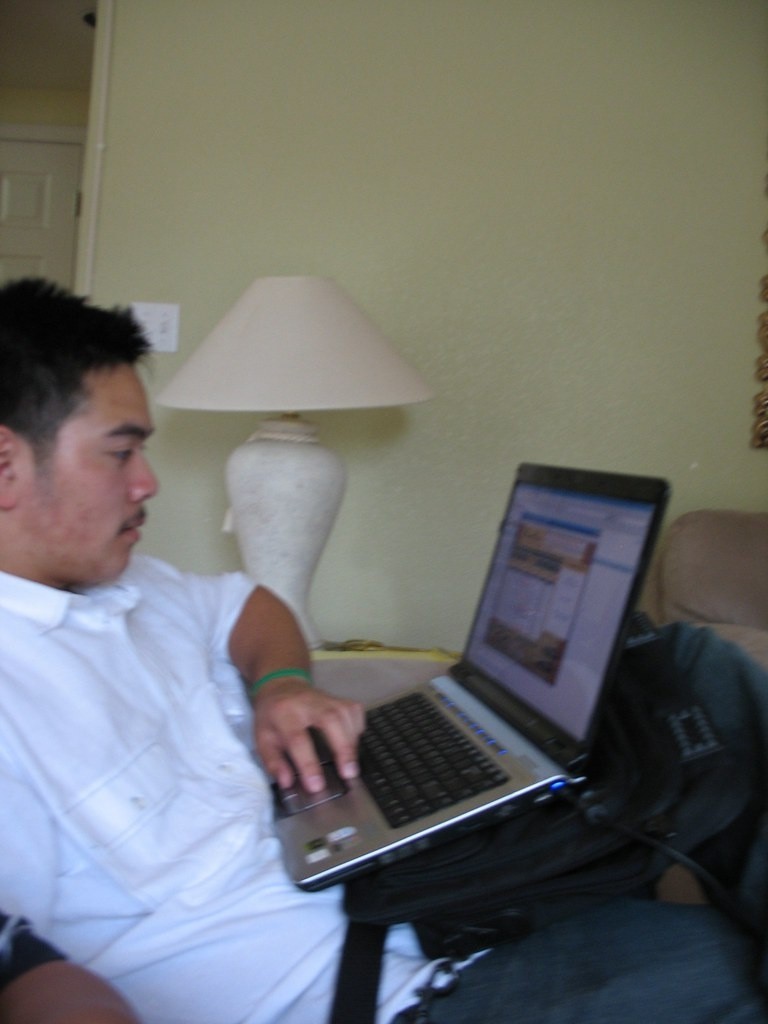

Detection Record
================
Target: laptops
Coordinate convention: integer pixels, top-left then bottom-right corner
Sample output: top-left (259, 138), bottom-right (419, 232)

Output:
top-left (261, 463), bottom-right (673, 891)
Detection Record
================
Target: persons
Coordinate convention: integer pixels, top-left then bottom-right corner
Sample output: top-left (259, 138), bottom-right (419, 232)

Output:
top-left (0, 276), bottom-right (768, 1024)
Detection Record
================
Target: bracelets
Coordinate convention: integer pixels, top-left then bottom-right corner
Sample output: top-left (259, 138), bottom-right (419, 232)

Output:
top-left (0, 911), bottom-right (68, 988)
top-left (249, 668), bottom-right (315, 699)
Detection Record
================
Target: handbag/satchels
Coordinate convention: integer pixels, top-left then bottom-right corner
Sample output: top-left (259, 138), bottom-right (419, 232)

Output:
top-left (341, 619), bottom-right (768, 928)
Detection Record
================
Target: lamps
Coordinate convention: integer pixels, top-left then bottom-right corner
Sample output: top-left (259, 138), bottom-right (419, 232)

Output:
top-left (157, 273), bottom-right (436, 651)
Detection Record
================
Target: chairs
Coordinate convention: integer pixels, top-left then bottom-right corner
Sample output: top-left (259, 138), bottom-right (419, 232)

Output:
top-left (656, 507), bottom-right (768, 637)
